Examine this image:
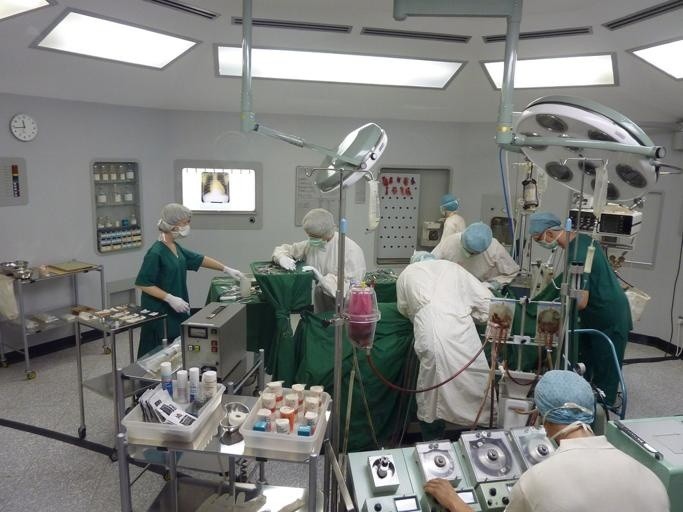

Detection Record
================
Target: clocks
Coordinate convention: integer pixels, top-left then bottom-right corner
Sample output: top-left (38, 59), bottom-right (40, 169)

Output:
top-left (9, 112), bottom-right (39, 142)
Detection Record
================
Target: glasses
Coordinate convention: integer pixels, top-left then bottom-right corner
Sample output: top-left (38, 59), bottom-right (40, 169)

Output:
top-left (535, 227), bottom-right (550, 243)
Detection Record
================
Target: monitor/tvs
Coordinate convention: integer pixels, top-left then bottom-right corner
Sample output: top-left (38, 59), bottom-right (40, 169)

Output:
top-left (180, 166), bottom-right (257, 216)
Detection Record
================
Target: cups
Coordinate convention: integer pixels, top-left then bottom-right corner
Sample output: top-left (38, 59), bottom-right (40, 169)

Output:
top-left (239, 277), bottom-right (251, 299)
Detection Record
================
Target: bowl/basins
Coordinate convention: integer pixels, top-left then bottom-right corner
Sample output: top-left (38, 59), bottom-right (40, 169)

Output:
top-left (0, 259), bottom-right (29, 275)
top-left (10, 269), bottom-right (33, 280)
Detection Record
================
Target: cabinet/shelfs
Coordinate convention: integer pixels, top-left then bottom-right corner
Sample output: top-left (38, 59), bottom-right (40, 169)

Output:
top-left (119, 335), bottom-right (271, 485)
top-left (69, 304), bottom-right (172, 462)
top-left (113, 392), bottom-right (334, 511)
top-left (86, 159), bottom-right (144, 254)
top-left (0, 255), bottom-right (112, 383)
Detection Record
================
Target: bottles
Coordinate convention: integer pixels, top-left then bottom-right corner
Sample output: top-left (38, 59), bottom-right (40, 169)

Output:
top-left (251, 379), bottom-right (323, 436)
top-left (161, 361), bottom-right (217, 405)
top-left (94, 163), bottom-right (138, 229)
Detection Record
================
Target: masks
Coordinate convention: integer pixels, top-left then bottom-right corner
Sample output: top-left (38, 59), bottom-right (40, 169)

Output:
top-left (170, 223), bottom-right (191, 240)
top-left (304, 235), bottom-right (328, 249)
top-left (462, 247), bottom-right (481, 259)
top-left (535, 239), bottom-right (559, 251)
top-left (439, 206), bottom-right (447, 217)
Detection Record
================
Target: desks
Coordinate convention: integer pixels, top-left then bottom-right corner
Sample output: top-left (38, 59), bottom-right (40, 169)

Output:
top-left (205, 275), bottom-right (276, 355)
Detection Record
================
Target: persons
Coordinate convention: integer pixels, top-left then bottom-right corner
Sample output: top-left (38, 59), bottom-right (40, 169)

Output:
top-left (528, 213), bottom-right (633, 411)
top-left (271, 208), bottom-right (367, 312)
top-left (422, 369), bottom-right (670, 512)
top-left (134, 203), bottom-right (246, 361)
top-left (395, 191), bottom-right (520, 429)
